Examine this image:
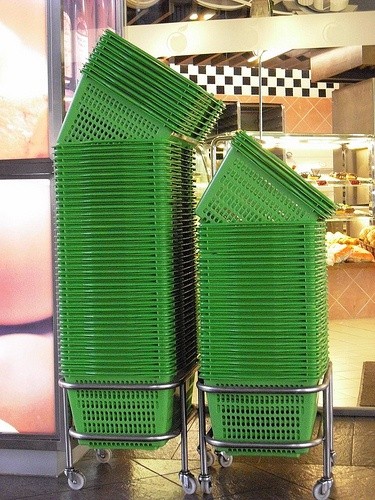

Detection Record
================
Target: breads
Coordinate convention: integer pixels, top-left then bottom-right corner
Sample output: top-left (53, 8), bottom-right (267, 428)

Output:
top-left (326, 225), bottom-right (375, 265)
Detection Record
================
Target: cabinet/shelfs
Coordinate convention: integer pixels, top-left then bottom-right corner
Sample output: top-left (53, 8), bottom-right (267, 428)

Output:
top-left (202, 130), bottom-right (375, 262)
